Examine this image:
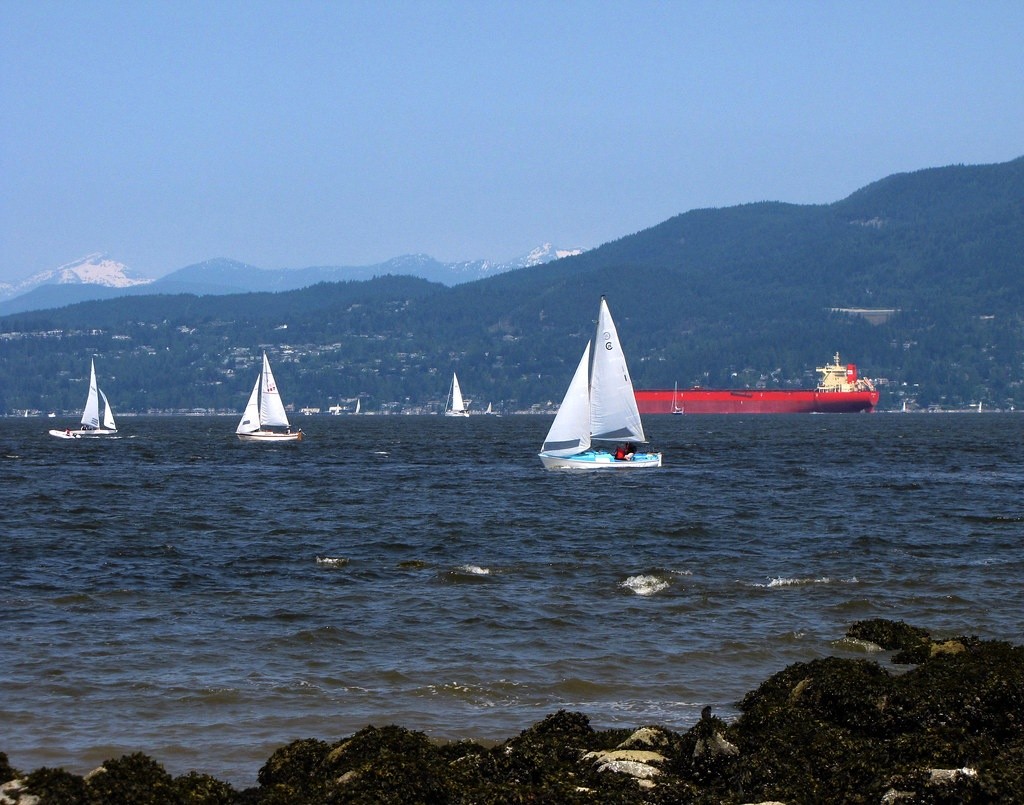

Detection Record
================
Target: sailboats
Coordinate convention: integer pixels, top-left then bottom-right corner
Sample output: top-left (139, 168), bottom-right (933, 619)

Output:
top-left (348, 399), bottom-right (363, 415)
top-left (537, 295), bottom-right (664, 472)
top-left (66, 358), bottom-right (118, 434)
top-left (331, 403), bottom-right (342, 415)
top-left (235, 349), bottom-right (302, 442)
top-left (671, 381), bottom-right (684, 414)
top-left (445, 372), bottom-right (469, 418)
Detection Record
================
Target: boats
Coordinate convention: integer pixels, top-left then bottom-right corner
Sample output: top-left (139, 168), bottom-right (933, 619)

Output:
top-left (305, 411), bottom-right (312, 416)
top-left (49, 428), bottom-right (82, 439)
top-left (635, 352), bottom-right (880, 414)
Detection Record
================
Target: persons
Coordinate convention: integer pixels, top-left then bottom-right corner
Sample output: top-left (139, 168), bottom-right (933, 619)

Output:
top-left (612, 444), bottom-right (625, 459)
top-left (621, 441), bottom-right (638, 460)
